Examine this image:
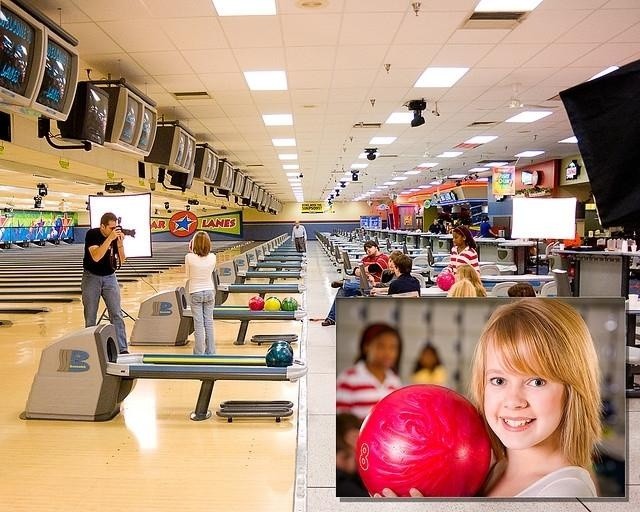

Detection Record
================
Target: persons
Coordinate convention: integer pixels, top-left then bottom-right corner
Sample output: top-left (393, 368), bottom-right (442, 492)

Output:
top-left (79, 211), bottom-right (128, 354)
top-left (321, 240), bottom-right (390, 326)
top-left (437, 225), bottom-right (482, 277)
top-left (371, 299), bottom-right (604, 497)
top-left (336, 413), bottom-right (370, 497)
top-left (429, 218), bottom-right (446, 234)
top-left (480, 215), bottom-right (497, 238)
top-left (369, 254), bottom-right (421, 296)
top-left (507, 283), bottom-right (535, 297)
top-left (291, 219), bottom-right (308, 252)
top-left (337, 322), bottom-right (404, 421)
top-left (184, 229), bottom-right (217, 355)
top-left (366, 249), bottom-right (402, 288)
top-left (447, 264), bottom-right (487, 297)
top-left (410, 343), bottom-right (445, 387)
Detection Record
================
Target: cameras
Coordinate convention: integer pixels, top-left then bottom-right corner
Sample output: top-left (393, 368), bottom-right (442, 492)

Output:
top-left (111, 216), bottom-right (136, 238)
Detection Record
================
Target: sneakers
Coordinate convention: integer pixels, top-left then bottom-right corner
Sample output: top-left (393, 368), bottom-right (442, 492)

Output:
top-left (331, 278), bottom-right (347, 288)
top-left (320, 317), bottom-right (335, 326)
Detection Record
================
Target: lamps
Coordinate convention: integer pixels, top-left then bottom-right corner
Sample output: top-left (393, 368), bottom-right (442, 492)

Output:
top-left (340, 181), bottom-right (346, 188)
top-left (299, 169), bottom-right (304, 178)
top-left (408, 99), bottom-right (426, 127)
top-left (335, 189), bottom-right (339, 196)
top-left (327, 199), bottom-right (331, 205)
top-left (351, 170), bottom-right (359, 181)
top-left (330, 194), bottom-right (334, 199)
top-left (365, 148), bottom-right (377, 160)
top-left (33, 195), bottom-right (46, 209)
top-left (36, 182), bottom-right (48, 197)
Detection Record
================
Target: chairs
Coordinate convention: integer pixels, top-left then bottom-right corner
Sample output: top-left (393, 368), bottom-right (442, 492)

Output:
top-left (313, 228), bottom-right (568, 297)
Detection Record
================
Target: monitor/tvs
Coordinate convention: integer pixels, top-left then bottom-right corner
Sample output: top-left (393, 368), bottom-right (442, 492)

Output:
top-left (136, 101), bottom-right (158, 159)
top-left (194, 142), bottom-right (284, 216)
top-left (30, 25), bottom-right (81, 124)
top-left (99, 86), bottom-right (144, 153)
top-left (146, 124), bottom-right (190, 173)
top-left (438, 191), bottom-right (458, 203)
top-left (57, 80), bottom-right (110, 149)
top-left (0, 0), bottom-right (46, 109)
top-left (184, 129), bottom-right (197, 173)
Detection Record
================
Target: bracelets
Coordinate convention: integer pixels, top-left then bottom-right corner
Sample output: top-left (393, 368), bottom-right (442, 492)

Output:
top-left (119, 245), bottom-right (123, 248)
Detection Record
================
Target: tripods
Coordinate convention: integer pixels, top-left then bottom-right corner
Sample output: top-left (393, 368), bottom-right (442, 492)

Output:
top-left (97, 305), bottom-right (135, 328)
